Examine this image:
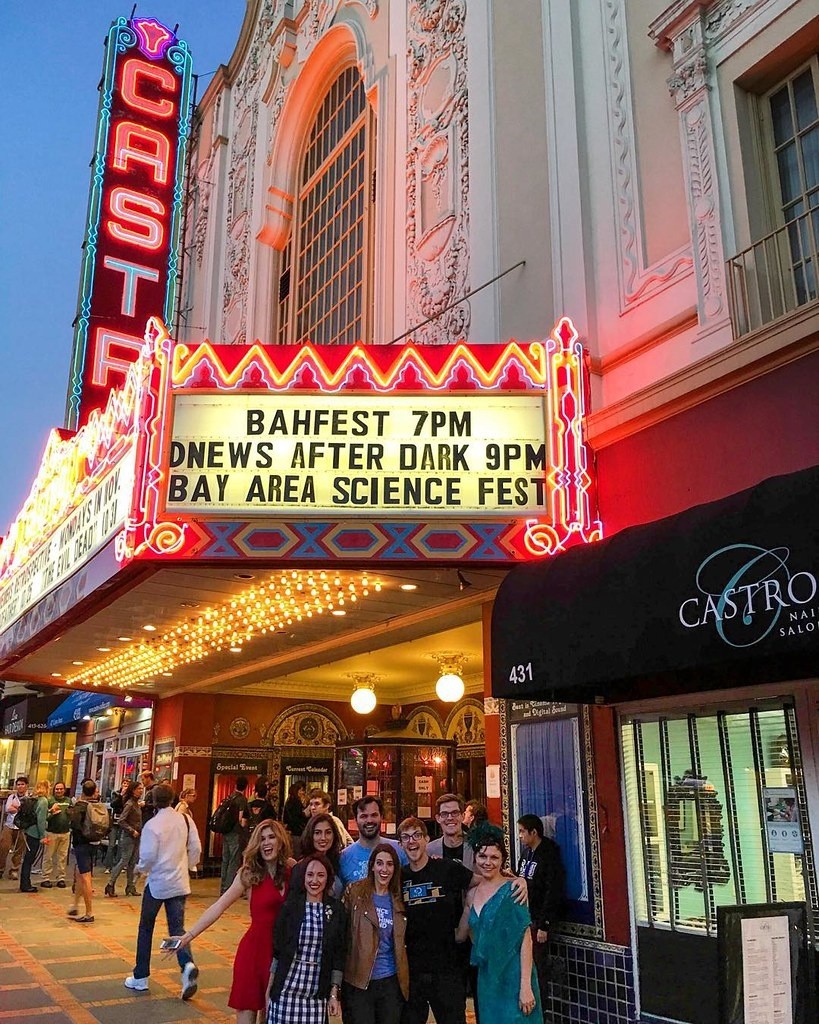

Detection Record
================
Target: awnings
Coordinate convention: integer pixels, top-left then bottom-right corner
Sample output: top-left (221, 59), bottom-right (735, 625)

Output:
top-left (46, 689), bottom-right (153, 728)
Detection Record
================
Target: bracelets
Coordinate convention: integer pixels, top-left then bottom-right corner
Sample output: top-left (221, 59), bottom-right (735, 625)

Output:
top-left (329, 994), bottom-right (338, 999)
top-left (188, 930), bottom-right (195, 939)
top-left (332, 986), bottom-right (341, 992)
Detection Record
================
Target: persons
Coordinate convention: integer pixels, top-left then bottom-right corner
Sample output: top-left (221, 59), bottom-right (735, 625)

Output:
top-left (337, 798), bottom-right (410, 895)
top-left (294, 813), bottom-right (343, 876)
top-left (264, 852), bottom-right (351, 1024)
top-left (0, 768), bottom-right (197, 922)
top-left (460, 799), bottom-right (489, 829)
top-left (124, 783), bottom-right (202, 1000)
top-left (160, 819), bottom-right (298, 1024)
top-left (340, 843), bottom-right (443, 1024)
top-left (308, 790), bottom-right (353, 848)
top-left (505, 814), bottom-right (564, 1024)
top-left (425, 794), bottom-right (484, 1024)
top-left (344, 816), bottom-right (528, 1024)
top-left (218, 777), bottom-right (278, 896)
top-left (455, 836), bottom-right (544, 1024)
top-left (282, 784), bottom-right (312, 836)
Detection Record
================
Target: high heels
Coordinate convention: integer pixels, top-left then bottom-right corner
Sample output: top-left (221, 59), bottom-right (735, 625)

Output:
top-left (125, 885), bottom-right (142, 896)
top-left (105, 884), bottom-right (118, 898)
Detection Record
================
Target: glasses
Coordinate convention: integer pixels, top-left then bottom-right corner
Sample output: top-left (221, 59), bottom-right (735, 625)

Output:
top-left (188, 794), bottom-right (197, 797)
top-left (398, 832), bottom-right (424, 843)
top-left (439, 810), bottom-right (462, 818)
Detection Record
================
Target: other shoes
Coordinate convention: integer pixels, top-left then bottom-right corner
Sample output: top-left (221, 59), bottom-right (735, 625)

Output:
top-left (121, 869), bottom-right (125, 872)
top-left (69, 910), bottom-right (78, 916)
top-left (26, 887), bottom-right (37, 892)
top-left (57, 881), bottom-right (66, 888)
top-left (41, 881), bottom-right (52, 888)
top-left (76, 915), bottom-right (94, 922)
top-left (104, 869), bottom-right (111, 874)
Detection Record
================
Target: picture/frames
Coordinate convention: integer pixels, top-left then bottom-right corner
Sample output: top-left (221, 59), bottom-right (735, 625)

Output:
top-left (717, 900), bottom-right (810, 1023)
top-left (679, 800), bottom-right (686, 831)
top-left (762, 787), bottom-right (804, 854)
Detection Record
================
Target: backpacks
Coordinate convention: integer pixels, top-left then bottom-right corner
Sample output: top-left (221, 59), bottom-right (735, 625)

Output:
top-left (13, 793), bottom-right (42, 830)
top-left (76, 800), bottom-right (110, 840)
top-left (209, 793), bottom-right (241, 834)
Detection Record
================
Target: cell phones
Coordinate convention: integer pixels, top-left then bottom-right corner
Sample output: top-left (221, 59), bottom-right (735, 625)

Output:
top-left (160, 940), bottom-right (181, 950)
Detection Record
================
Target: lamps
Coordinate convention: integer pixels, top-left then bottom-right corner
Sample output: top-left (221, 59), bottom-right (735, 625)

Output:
top-left (347, 672), bottom-right (380, 714)
top-left (431, 652), bottom-right (469, 703)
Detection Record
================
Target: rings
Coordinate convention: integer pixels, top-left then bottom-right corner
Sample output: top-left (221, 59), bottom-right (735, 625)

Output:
top-left (531, 1005), bottom-right (534, 1008)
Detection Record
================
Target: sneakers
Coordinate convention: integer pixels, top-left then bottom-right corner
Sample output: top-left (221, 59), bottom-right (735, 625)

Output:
top-left (125, 975), bottom-right (148, 992)
top-left (181, 961), bottom-right (198, 1000)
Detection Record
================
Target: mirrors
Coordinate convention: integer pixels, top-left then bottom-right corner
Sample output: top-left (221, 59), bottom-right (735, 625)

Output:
top-left (664, 768), bottom-right (731, 894)
